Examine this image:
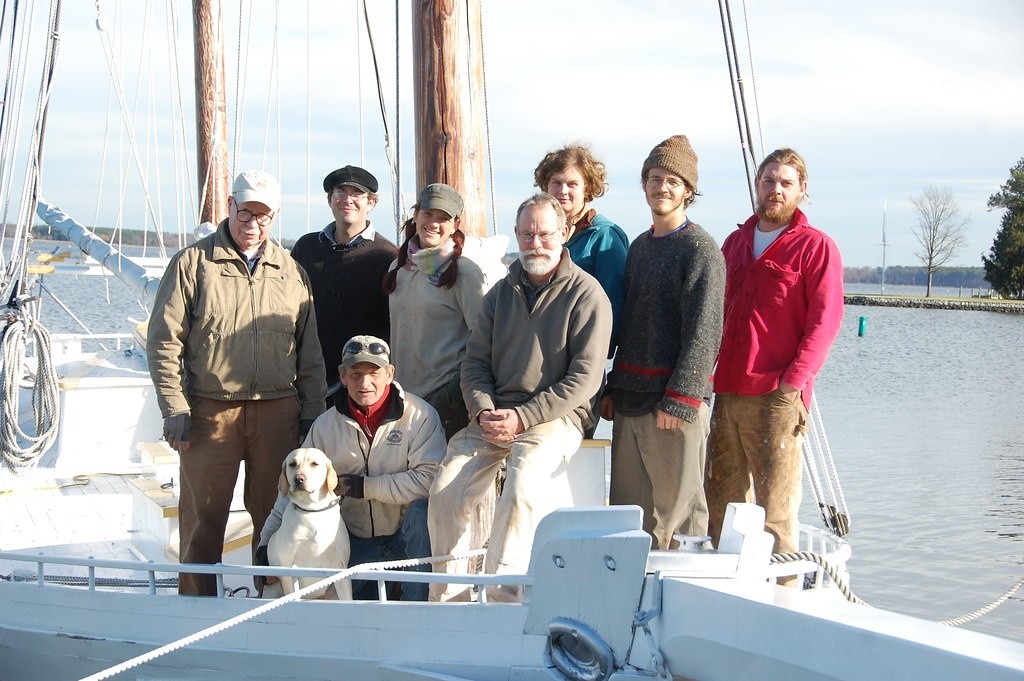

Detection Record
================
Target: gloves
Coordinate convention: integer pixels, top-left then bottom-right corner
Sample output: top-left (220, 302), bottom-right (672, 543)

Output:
top-left (337, 472), bottom-right (364, 500)
top-left (255, 546), bottom-right (268, 566)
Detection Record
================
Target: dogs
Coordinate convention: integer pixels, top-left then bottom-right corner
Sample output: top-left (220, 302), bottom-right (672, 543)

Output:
top-left (260, 446), bottom-right (357, 601)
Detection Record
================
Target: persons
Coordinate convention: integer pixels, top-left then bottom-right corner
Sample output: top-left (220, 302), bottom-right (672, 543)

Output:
top-left (146, 171), bottom-right (327, 599)
top-left (599, 135), bottom-right (726, 549)
top-left (383, 183), bottom-right (488, 440)
top-left (704, 149), bottom-right (845, 587)
top-left (534, 146), bottom-right (630, 441)
top-left (289, 165), bottom-right (399, 386)
top-left (255, 335), bottom-right (447, 602)
top-left (428, 193), bottom-right (613, 603)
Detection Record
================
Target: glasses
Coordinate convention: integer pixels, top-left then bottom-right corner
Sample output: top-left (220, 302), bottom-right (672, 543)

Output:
top-left (332, 188), bottom-right (373, 202)
top-left (518, 225), bottom-right (563, 243)
top-left (644, 176), bottom-right (687, 190)
top-left (343, 342), bottom-right (388, 357)
top-left (232, 195), bottom-right (275, 228)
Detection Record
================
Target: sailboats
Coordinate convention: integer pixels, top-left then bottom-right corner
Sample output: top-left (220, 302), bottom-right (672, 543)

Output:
top-left (0, 0), bottom-right (852, 657)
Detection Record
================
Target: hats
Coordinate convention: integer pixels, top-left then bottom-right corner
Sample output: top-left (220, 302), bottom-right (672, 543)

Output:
top-left (418, 183), bottom-right (464, 218)
top-left (642, 134), bottom-right (698, 191)
top-left (340, 335), bottom-right (390, 367)
top-left (323, 165), bottom-right (377, 196)
top-left (232, 171), bottom-right (281, 212)
